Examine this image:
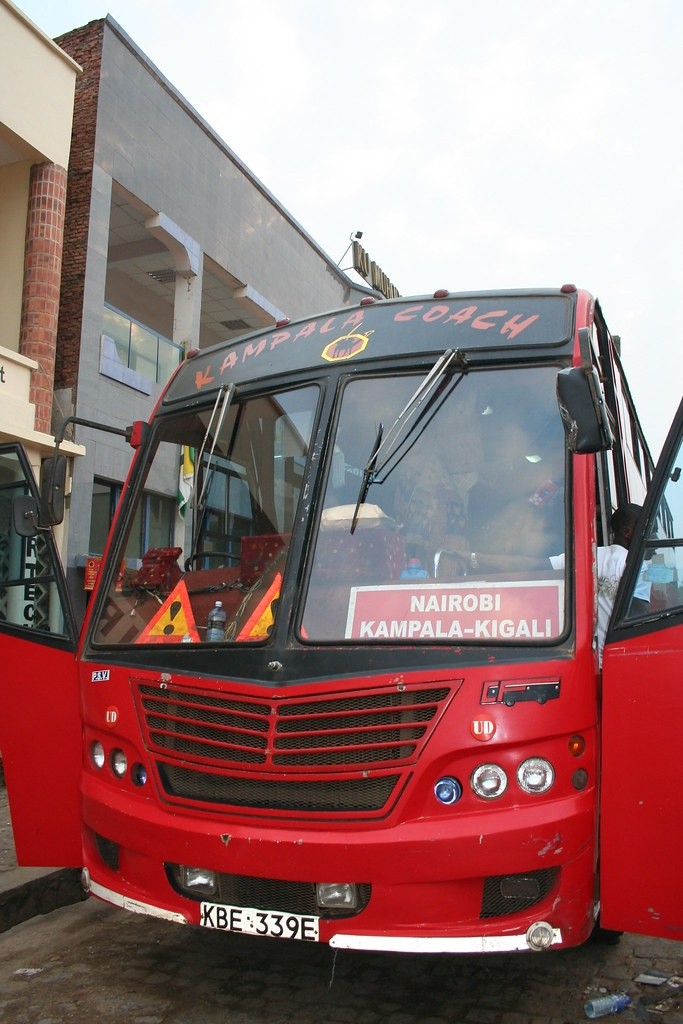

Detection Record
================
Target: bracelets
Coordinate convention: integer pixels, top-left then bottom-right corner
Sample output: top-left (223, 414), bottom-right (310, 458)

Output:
top-left (470, 552), bottom-right (479, 570)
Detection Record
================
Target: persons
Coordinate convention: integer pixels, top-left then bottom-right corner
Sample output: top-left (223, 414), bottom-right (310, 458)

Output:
top-left (455, 503), bottom-right (660, 671)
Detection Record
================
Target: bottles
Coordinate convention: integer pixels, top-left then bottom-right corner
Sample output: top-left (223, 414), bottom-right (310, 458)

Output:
top-left (400, 558), bottom-right (428, 579)
top-left (207, 601), bottom-right (226, 641)
top-left (584, 995), bottom-right (631, 1018)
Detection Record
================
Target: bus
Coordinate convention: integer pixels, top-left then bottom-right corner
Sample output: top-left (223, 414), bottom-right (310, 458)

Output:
top-left (2, 284), bottom-right (683, 958)
